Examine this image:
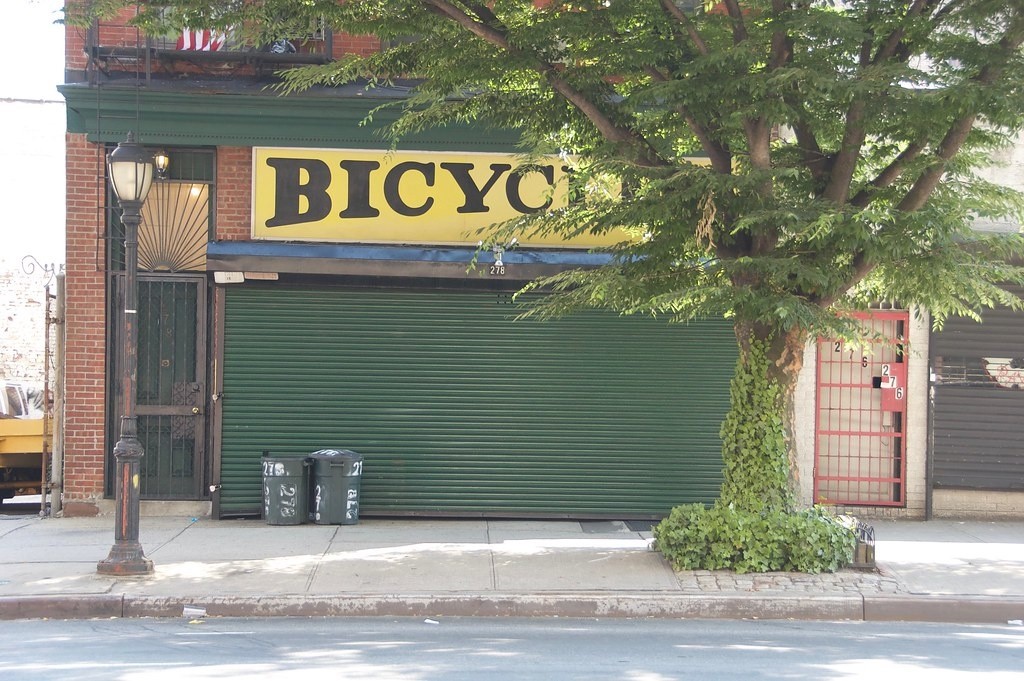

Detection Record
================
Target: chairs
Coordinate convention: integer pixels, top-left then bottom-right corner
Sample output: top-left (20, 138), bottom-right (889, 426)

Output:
top-left (176, 6), bottom-right (230, 53)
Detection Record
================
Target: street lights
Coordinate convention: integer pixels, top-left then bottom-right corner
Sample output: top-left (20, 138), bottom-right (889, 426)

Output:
top-left (97, 134), bottom-right (156, 575)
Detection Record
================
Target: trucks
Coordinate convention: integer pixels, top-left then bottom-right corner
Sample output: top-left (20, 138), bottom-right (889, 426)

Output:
top-left (1, 417), bottom-right (64, 509)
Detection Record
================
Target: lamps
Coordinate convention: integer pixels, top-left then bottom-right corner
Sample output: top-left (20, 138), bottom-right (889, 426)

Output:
top-left (155, 149), bottom-right (169, 180)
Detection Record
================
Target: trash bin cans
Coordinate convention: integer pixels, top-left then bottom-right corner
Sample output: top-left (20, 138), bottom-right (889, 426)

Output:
top-left (260, 456), bottom-right (313, 527)
top-left (309, 448), bottom-right (364, 525)
